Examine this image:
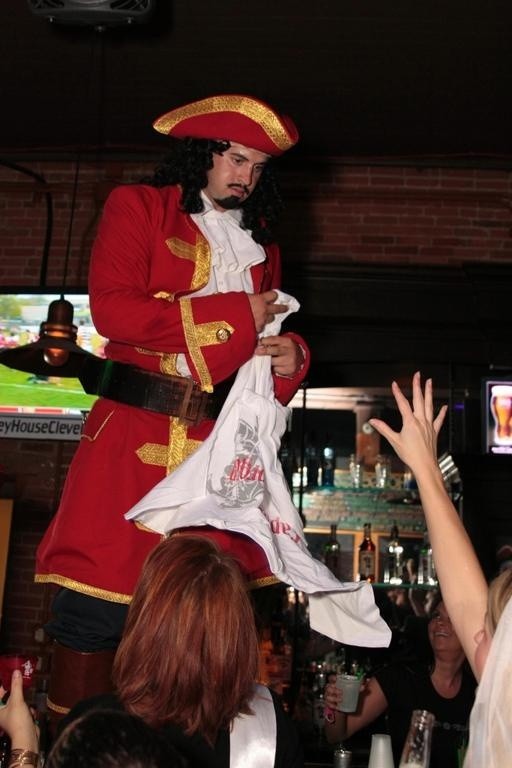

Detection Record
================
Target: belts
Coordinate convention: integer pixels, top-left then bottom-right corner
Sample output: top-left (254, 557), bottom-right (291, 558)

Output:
top-left (95, 358), bottom-right (227, 422)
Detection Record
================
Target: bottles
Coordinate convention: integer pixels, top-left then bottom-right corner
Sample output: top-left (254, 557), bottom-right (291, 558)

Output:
top-left (381, 519), bottom-right (405, 585)
top-left (294, 660), bottom-right (326, 739)
top-left (359, 521), bottom-right (376, 584)
top-left (334, 750), bottom-right (351, 766)
top-left (418, 524), bottom-right (438, 585)
top-left (321, 525), bottom-right (343, 577)
top-left (285, 444), bottom-right (337, 492)
top-left (401, 710), bottom-right (434, 768)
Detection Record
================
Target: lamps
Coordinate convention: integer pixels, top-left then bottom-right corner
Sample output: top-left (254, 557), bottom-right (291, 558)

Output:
top-left (0, 147), bottom-right (107, 378)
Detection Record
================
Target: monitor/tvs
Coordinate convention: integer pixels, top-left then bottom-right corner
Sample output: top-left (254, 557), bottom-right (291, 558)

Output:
top-left (460, 361), bottom-right (512, 468)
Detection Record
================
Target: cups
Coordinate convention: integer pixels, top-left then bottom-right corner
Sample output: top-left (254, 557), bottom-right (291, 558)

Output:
top-left (349, 453), bottom-right (366, 487)
top-left (337, 673), bottom-right (359, 714)
top-left (367, 732), bottom-right (394, 768)
top-left (0, 653), bottom-right (39, 689)
top-left (374, 452), bottom-right (392, 488)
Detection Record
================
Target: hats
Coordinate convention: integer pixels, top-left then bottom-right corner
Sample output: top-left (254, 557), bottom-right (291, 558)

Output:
top-left (150, 92), bottom-right (303, 157)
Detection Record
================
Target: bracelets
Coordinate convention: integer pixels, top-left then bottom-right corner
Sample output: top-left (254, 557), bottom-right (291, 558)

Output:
top-left (9, 749), bottom-right (38, 768)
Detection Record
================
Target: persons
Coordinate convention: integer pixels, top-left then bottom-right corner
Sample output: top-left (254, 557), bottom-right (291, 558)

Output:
top-left (0, 534), bottom-right (305, 768)
top-left (323, 594), bottom-right (476, 768)
top-left (0, 316), bottom-right (109, 386)
top-left (32, 87), bottom-right (314, 745)
top-left (368, 370), bottom-right (512, 768)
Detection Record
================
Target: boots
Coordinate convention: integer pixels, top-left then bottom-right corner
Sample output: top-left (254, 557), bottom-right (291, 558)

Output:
top-left (42, 639), bottom-right (112, 752)
